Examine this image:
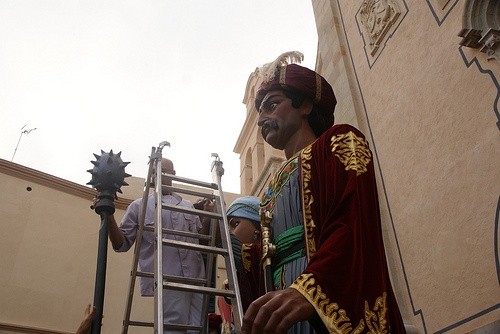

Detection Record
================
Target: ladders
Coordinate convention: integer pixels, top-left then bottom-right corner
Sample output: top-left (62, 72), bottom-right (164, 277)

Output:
top-left (121, 142), bottom-right (243, 334)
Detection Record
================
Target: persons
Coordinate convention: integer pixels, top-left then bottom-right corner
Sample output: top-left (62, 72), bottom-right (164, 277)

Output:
top-left (94, 158), bottom-right (211, 334)
top-left (76, 304), bottom-right (94, 334)
top-left (242, 65), bottom-right (408, 334)
top-left (227, 196), bottom-right (262, 314)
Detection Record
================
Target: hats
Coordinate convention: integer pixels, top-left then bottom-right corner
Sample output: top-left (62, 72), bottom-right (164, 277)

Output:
top-left (227, 196), bottom-right (261, 221)
top-left (253, 50), bottom-right (338, 130)
